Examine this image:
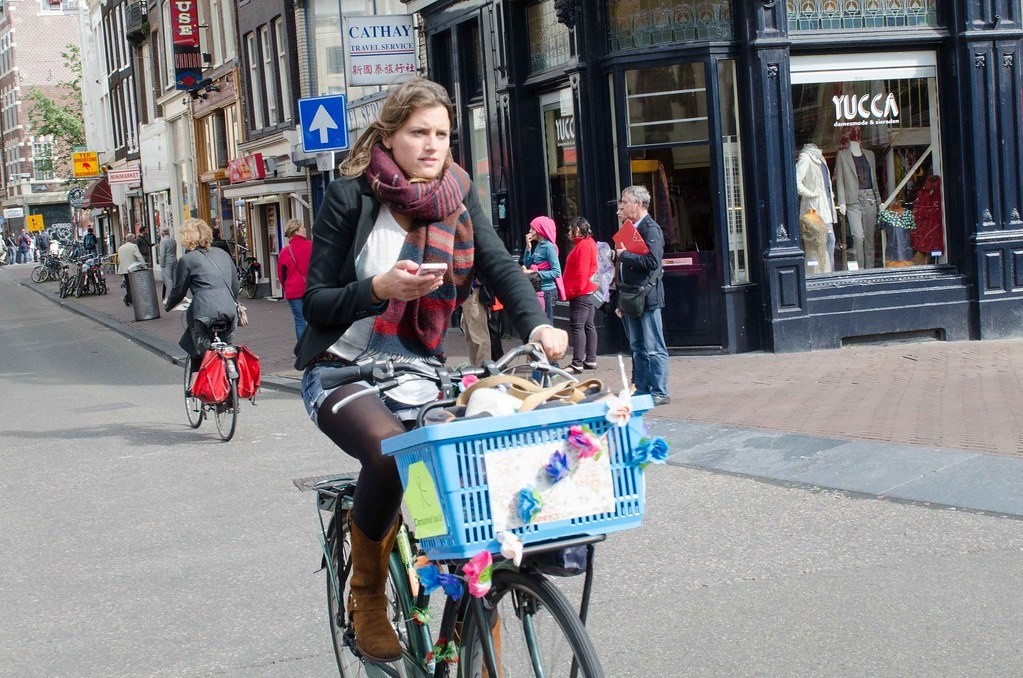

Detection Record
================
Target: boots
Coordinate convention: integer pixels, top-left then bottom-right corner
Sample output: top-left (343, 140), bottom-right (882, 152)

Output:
top-left (347, 508), bottom-right (402, 662)
top-left (456, 616), bottom-right (502, 678)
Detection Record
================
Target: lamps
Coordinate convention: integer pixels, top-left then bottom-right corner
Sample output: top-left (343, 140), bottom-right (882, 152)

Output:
top-left (190, 85), bottom-right (219, 100)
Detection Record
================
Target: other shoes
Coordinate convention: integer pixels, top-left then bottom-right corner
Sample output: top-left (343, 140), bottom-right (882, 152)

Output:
top-left (583, 361), bottom-right (597, 368)
top-left (654, 396), bottom-right (670, 405)
top-left (562, 364), bottom-right (583, 373)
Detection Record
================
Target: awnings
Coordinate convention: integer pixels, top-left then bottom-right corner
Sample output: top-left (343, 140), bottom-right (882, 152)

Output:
top-left (82, 177), bottom-right (113, 210)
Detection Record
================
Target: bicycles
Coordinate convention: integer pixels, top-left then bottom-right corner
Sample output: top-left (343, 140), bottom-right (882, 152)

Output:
top-left (165, 297), bottom-right (243, 441)
top-left (229, 242), bottom-right (262, 299)
top-left (292, 342), bottom-right (607, 678)
top-left (32, 252), bottom-right (65, 283)
top-left (55, 238), bottom-right (110, 299)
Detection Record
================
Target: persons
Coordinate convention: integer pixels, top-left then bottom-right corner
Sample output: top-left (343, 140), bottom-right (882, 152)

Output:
top-left (230, 223), bottom-right (247, 253)
top-left (563, 218), bottom-right (598, 374)
top-left (158, 228), bottom-right (177, 301)
top-left (293, 82), bottom-right (567, 678)
top-left (462, 287), bottom-right (507, 371)
top-left (118, 227), bottom-right (151, 306)
top-left (799, 209), bottom-right (830, 274)
top-left (212, 229), bottom-right (231, 254)
top-left (212, 218), bottom-right (220, 229)
top-left (880, 203), bottom-right (916, 262)
top-left (83, 225), bottom-right (97, 259)
top-left (836, 141), bottom-right (881, 269)
top-left (277, 219), bottom-right (313, 340)
top-left (522, 216), bottom-right (560, 379)
top-left (163, 219), bottom-right (240, 414)
top-left (796, 143), bottom-right (837, 272)
top-left (615, 187), bottom-right (670, 406)
top-left (0, 229), bottom-right (51, 266)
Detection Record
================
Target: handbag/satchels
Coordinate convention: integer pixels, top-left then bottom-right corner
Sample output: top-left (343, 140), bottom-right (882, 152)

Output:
top-left (191, 351), bottom-right (231, 405)
top-left (619, 283), bottom-right (646, 316)
top-left (232, 344), bottom-right (261, 400)
top-left (85, 234), bottom-right (95, 249)
top-left (236, 304), bottom-right (248, 326)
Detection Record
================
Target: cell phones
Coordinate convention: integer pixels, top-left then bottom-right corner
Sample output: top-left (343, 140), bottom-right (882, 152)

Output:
top-left (415, 263), bottom-right (447, 276)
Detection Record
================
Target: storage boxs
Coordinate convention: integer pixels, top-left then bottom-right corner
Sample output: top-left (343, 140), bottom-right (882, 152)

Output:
top-left (381, 393), bottom-right (652, 560)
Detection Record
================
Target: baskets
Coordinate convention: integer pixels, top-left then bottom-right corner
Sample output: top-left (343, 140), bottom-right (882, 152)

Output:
top-left (380, 393), bottom-right (655, 560)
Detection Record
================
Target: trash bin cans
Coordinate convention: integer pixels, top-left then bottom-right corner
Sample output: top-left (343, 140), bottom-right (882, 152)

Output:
top-left (128, 262), bottom-right (161, 322)
